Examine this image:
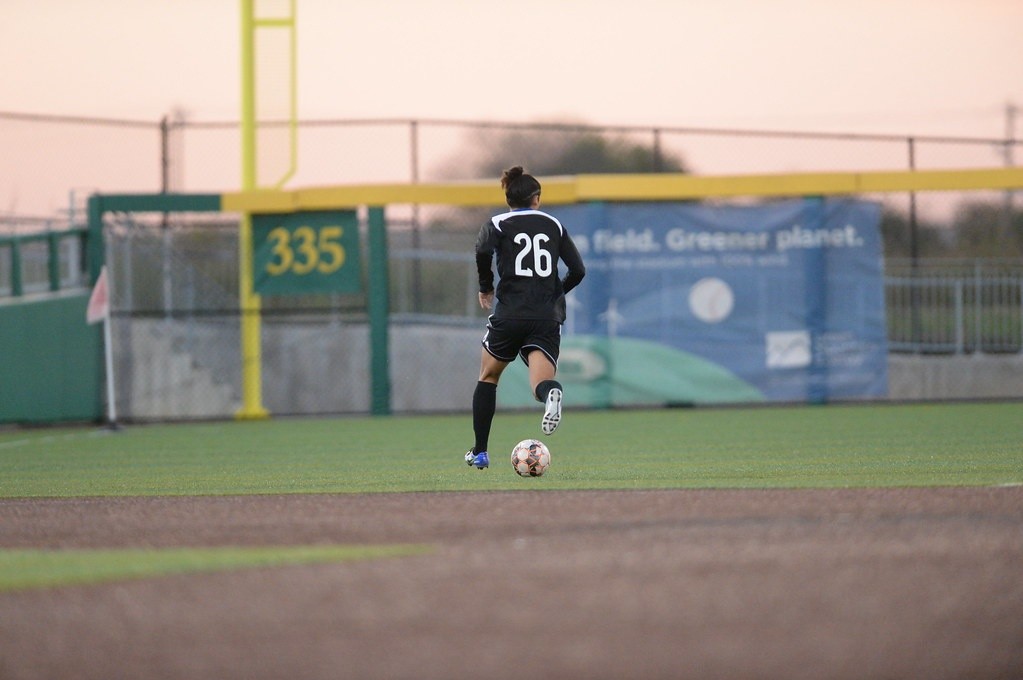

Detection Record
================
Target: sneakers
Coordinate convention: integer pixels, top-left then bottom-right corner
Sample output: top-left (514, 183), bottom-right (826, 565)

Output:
top-left (542, 388), bottom-right (562, 435)
top-left (465, 447), bottom-right (489, 470)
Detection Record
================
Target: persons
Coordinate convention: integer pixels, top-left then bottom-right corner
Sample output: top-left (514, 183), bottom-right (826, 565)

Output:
top-left (463, 165), bottom-right (586, 471)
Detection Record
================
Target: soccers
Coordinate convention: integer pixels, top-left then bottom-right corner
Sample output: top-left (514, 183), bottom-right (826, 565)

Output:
top-left (510, 438), bottom-right (552, 477)
